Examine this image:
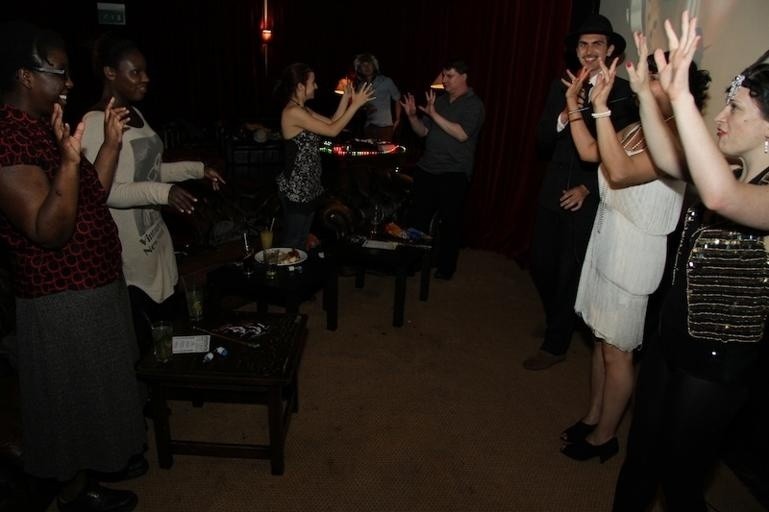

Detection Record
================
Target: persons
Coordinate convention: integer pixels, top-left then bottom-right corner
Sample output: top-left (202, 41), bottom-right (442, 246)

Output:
top-left (523, 12), bottom-right (628, 369)
top-left (404, 58), bottom-right (486, 280)
top-left (559, 51), bottom-right (712, 463)
top-left (1, 29), bottom-right (146, 511)
top-left (78, 34), bottom-right (224, 420)
top-left (613, 8), bottom-right (769, 511)
top-left (354, 53), bottom-right (401, 158)
top-left (271, 58), bottom-right (375, 301)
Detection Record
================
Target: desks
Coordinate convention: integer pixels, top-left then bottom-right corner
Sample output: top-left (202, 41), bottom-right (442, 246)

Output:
top-left (323, 222), bottom-right (435, 330)
top-left (210, 248), bottom-right (339, 332)
top-left (141, 309), bottom-right (310, 477)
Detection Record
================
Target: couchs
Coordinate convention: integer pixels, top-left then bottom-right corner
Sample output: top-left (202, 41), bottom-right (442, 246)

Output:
top-left (164, 175), bottom-right (356, 266)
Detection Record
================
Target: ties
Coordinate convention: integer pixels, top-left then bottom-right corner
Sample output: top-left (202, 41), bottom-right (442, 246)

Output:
top-left (582, 83), bottom-right (593, 107)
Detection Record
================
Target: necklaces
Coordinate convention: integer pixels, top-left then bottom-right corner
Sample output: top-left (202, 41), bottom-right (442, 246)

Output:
top-left (597, 113), bottom-right (675, 235)
top-left (290, 98), bottom-right (309, 112)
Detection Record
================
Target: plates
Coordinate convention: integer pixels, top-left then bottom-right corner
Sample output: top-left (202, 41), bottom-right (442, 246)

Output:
top-left (255, 247), bottom-right (308, 266)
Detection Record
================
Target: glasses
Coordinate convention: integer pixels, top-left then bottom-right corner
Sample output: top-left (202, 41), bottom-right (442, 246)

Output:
top-left (22, 65), bottom-right (72, 81)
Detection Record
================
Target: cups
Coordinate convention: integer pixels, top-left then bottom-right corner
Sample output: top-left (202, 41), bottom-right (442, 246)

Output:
top-left (185, 290), bottom-right (206, 323)
top-left (260, 228), bottom-right (273, 249)
top-left (263, 249), bottom-right (279, 279)
top-left (242, 245), bottom-right (255, 277)
top-left (152, 320), bottom-right (172, 364)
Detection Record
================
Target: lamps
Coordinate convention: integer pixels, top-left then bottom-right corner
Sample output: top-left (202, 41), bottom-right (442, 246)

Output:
top-left (335, 76), bottom-right (354, 96)
top-left (430, 70), bottom-right (447, 91)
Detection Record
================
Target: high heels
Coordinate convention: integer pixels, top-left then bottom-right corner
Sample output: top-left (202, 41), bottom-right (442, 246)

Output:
top-left (561, 421), bottom-right (599, 442)
top-left (560, 436), bottom-right (618, 464)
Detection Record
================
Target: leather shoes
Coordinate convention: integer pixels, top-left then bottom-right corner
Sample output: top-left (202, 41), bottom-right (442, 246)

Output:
top-left (530, 324), bottom-right (549, 339)
top-left (435, 267), bottom-right (456, 280)
top-left (84, 454), bottom-right (147, 480)
top-left (524, 350), bottom-right (565, 370)
top-left (58, 484), bottom-right (137, 512)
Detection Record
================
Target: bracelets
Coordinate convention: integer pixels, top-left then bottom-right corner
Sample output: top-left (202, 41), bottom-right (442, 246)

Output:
top-left (592, 110), bottom-right (612, 118)
top-left (568, 109), bottom-right (583, 123)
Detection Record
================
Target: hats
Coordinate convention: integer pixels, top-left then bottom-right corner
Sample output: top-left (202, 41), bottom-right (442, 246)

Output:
top-left (562, 16), bottom-right (626, 66)
